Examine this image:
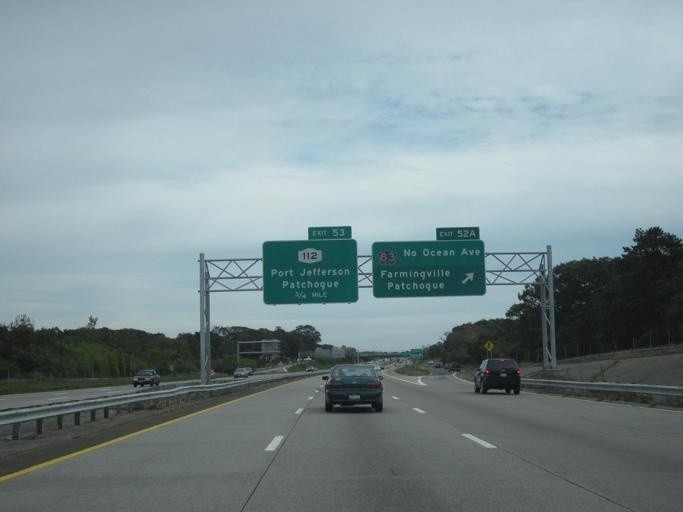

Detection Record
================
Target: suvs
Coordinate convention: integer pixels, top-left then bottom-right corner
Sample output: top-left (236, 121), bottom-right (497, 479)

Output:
top-left (473, 359), bottom-right (521, 396)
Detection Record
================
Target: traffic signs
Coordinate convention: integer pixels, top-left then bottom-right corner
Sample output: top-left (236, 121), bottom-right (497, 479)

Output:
top-left (308, 226), bottom-right (352, 240)
top-left (411, 349), bottom-right (423, 359)
top-left (373, 239), bottom-right (485, 298)
top-left (436, 227), bottom-right (479, 240)
top-left (262, 239), bottom-right (358, 306)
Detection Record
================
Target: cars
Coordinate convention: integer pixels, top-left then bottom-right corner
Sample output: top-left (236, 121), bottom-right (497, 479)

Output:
top-left (210, 369), bottom-right (216, 378)
top-left (234, 368), bottom-right (248, 378)
top-left (433, 361), bottom-right (461, 373)
top-left (132, 369), bottom-right (160, 387)
top-left (320, 363), bottom-right (384, 413)
top-left (305, 366), bottom-right (314, 372)
